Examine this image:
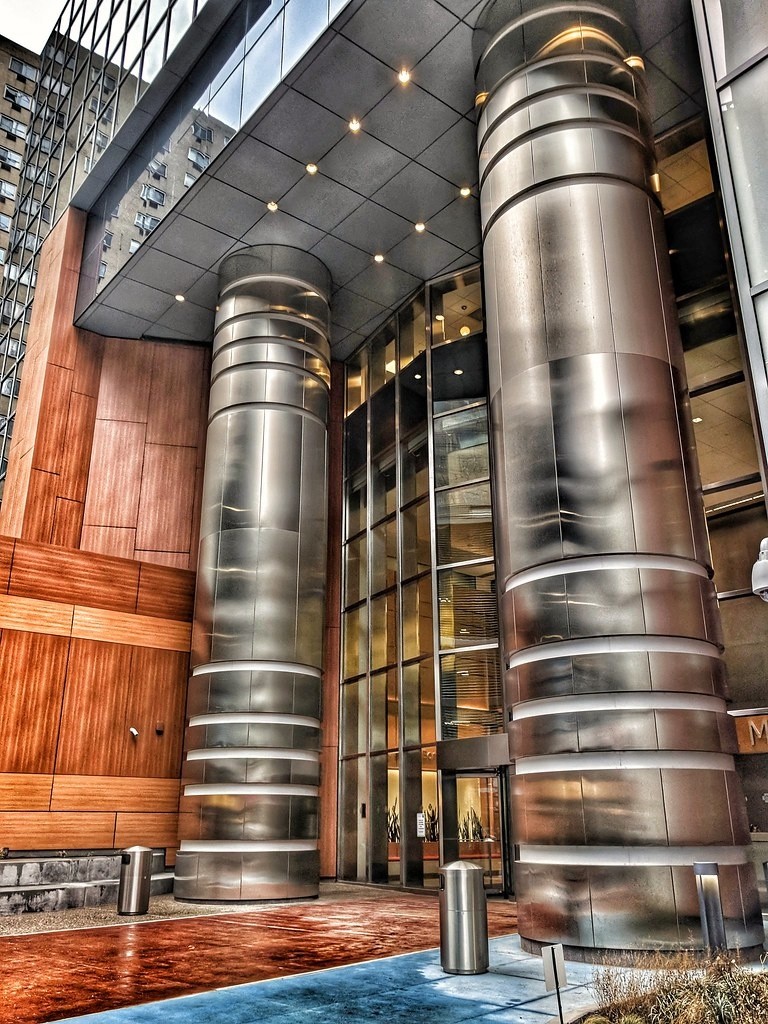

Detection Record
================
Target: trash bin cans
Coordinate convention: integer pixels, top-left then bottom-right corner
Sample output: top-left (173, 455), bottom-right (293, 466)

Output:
top-left (436, 860), bottom-right (489, 975)
top-left (117, 846), bottom-right (153, 916)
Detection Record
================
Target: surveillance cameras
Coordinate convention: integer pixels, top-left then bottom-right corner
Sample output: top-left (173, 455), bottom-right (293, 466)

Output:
top-left (752, 537), bottom-right (768, 602)
